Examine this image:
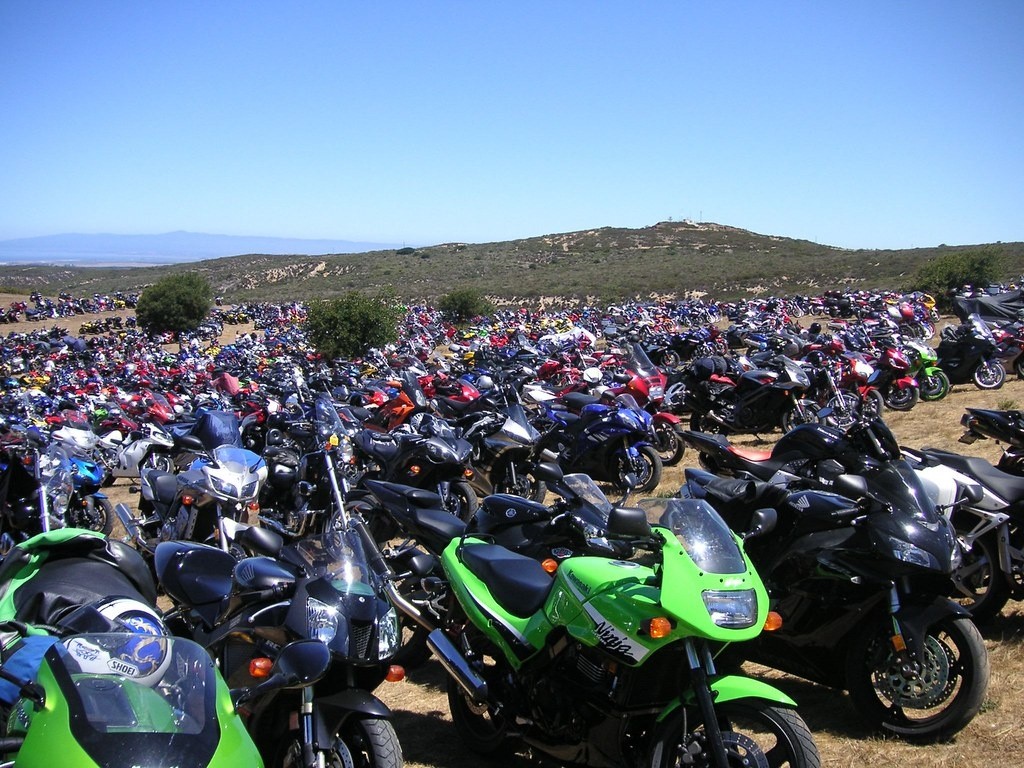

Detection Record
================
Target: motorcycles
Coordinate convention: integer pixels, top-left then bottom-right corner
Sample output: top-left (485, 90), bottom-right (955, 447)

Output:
top-left (0, 279), bottom-right (1024, 768)
